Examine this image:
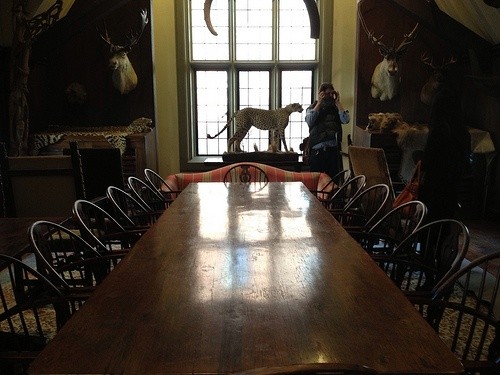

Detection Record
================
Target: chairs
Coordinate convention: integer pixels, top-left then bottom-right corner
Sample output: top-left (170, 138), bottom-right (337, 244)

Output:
top-left (315, 169), bottom-right (500, 375)
top-left (0, 168), bottom-right (180, 375)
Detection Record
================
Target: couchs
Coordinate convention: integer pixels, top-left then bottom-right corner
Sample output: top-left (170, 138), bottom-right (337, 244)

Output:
top-left (160, 162), bottom-right (338, 198)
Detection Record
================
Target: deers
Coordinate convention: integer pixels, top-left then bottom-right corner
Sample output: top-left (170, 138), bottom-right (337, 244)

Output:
top-left (416, 47), bottom-right (500, 108)
top-left (94, 6), bottom-right (150, 96)
top-left (356, 0), bottom-right (426, 103)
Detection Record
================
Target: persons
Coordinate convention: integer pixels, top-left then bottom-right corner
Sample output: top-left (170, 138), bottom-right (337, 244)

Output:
top-left (305, 82), bottom-right (350, 187)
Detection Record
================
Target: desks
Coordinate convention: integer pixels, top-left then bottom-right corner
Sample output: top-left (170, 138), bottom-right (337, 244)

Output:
top-left (28, 125), bottom-right (146, 204)
top-left (27, 181), bottom-right (464, 375)
top-left (355, 125), bottom-right (495, 163)
